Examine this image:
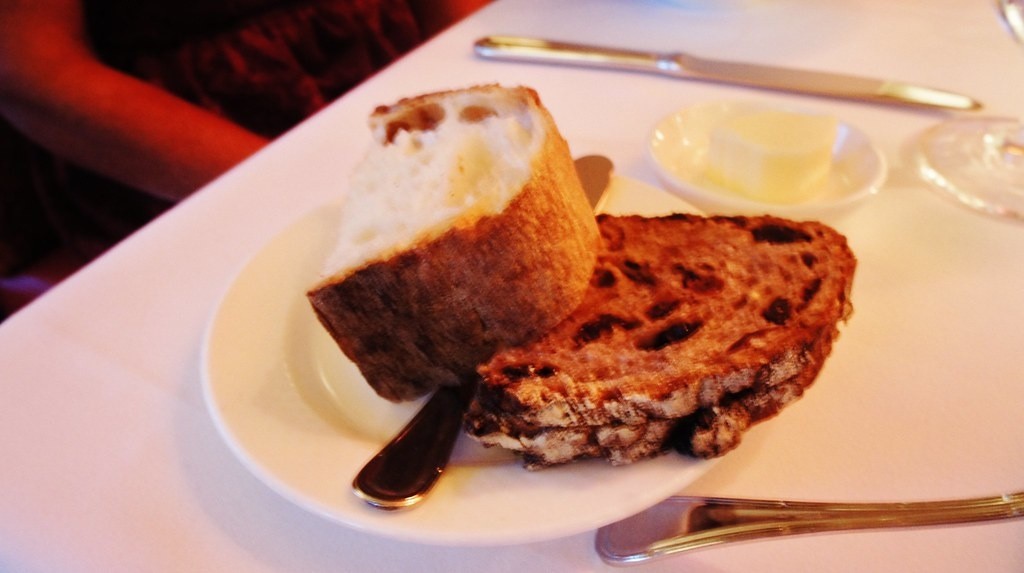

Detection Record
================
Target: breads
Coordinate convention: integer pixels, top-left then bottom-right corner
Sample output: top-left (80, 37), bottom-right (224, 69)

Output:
top-left (302, 87), bottom-right (602, 404)
top-left (460, 214), bottom-right (858, 465)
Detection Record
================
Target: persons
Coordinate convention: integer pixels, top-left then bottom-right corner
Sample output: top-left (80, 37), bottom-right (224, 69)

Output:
top-left (0, 0), bottom-right (495, 200)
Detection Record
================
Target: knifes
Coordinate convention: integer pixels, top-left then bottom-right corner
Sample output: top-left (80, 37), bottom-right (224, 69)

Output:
top-left (351, 154), bottom-right (617, 509)
top-left (473, 35), bottom-right (984, 122)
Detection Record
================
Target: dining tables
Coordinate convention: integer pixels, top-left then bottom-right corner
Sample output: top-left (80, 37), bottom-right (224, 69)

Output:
top-left (0, 0), bottom-right (1024, 572)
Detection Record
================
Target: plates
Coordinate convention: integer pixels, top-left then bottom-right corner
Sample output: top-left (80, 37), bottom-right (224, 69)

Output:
top-left (652, 94), bottom-right (888, 220)
top-left (201, 168), bottom-right (741, 547)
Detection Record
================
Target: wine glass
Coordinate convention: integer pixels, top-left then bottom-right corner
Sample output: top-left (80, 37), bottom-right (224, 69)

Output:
top-left (911, 0), bottom-right (1024, 218)
